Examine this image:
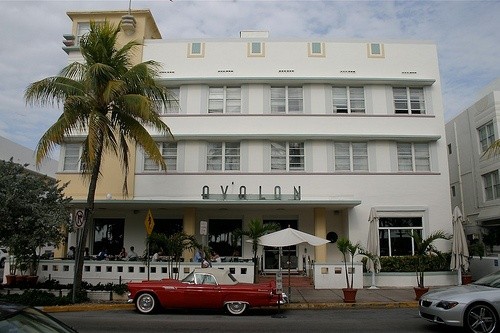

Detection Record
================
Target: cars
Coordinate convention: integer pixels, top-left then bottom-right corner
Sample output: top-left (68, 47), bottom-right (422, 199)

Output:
top-left (124, 267), bottom-right (290, 316)
top-left (417, 270), bottom-right (500, 333)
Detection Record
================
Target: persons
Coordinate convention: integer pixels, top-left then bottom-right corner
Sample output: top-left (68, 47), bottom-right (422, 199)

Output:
top-left (152, 247), bottom-right (163, 262)
top-left (119, 248), bottom-right (128, 258)
top-left (67, 246), bottom-right (75, 259)
top-left (211, 250), bottom-right (219, 262)
top-left (85, 247), bottom-right (90, 256)
top-left (126, 246), bottom-right (138, 261)
top-left (194, 248), bottom-right (202, 262)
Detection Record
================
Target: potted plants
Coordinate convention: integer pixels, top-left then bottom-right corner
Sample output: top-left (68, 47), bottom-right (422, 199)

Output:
top-left (338, 239), bottom-right (379, 303)
top-left (461, 255), bottom-right (473, 286)
top-left (407, 230), bottom-right (453, 300)
top-left (5, 245), bottom-right (39, 287)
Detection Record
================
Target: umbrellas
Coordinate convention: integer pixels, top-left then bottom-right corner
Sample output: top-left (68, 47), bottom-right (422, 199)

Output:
top-left (246, 225), bottom-right (331, 285)
top-left (449, 206), bottom-right (469, 286)
top-left (366, 207), bottom-right (382, 285)
